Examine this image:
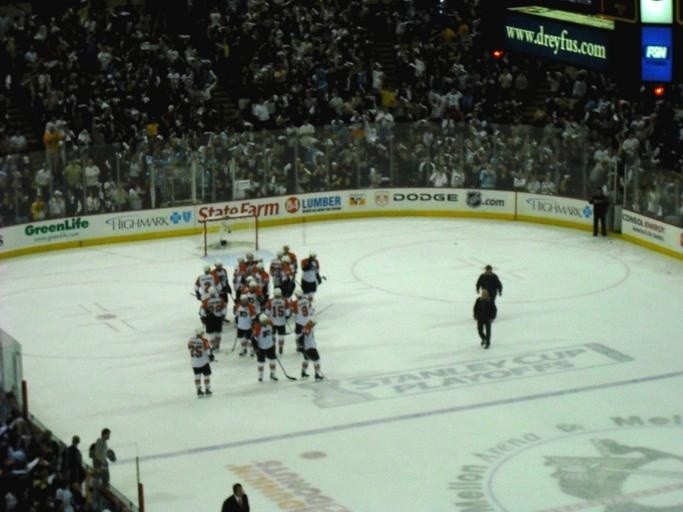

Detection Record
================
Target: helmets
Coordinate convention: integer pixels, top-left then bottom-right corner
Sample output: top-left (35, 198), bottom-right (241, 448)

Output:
top-left (202, 244), bottom-right (318, 323)
top-left (195, 326), bottom-right (206, 336)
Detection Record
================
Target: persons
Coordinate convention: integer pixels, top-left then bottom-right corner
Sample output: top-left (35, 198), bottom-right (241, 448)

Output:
top-left (185, 244), bottom-right (326, 397)
top-left (221, 483), bottom-right (250, 512)
top-left (0, 390), bottom-right (117, 512)
top-left (476, 265), bottom-right (502, 305)
top-left (472, 289), bottom-right (496, 348)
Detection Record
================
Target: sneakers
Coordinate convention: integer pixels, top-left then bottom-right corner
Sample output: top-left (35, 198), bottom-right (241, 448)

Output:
top-left (258, 377), bottom-right (263, 382)
top-left (315, 373), bottom-right (323, 380)
top-left (239, 348), bottom-right (247, 356)
top-left (278, 347), bottom-right (283, 353)
top-left (270, 375), bottom-right (278, 381)
top-left (212, 344), bottom-right (220, 350)
top-left (196, 388), bottom-right (212, 395)
top-left (480, 342), bottom-right (490, 349)
top-left (301, 370), bottom-right (309, 377)
top-left (250, 350), bottom-right (255, 357)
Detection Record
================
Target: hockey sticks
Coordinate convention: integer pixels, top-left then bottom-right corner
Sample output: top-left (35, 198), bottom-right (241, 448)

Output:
top-left (276, 356), bottom-right (297, 380)
top-left (225, 336), bottom-right (238, 355)
top-left (316, 275), bottom-right (327, 287)
